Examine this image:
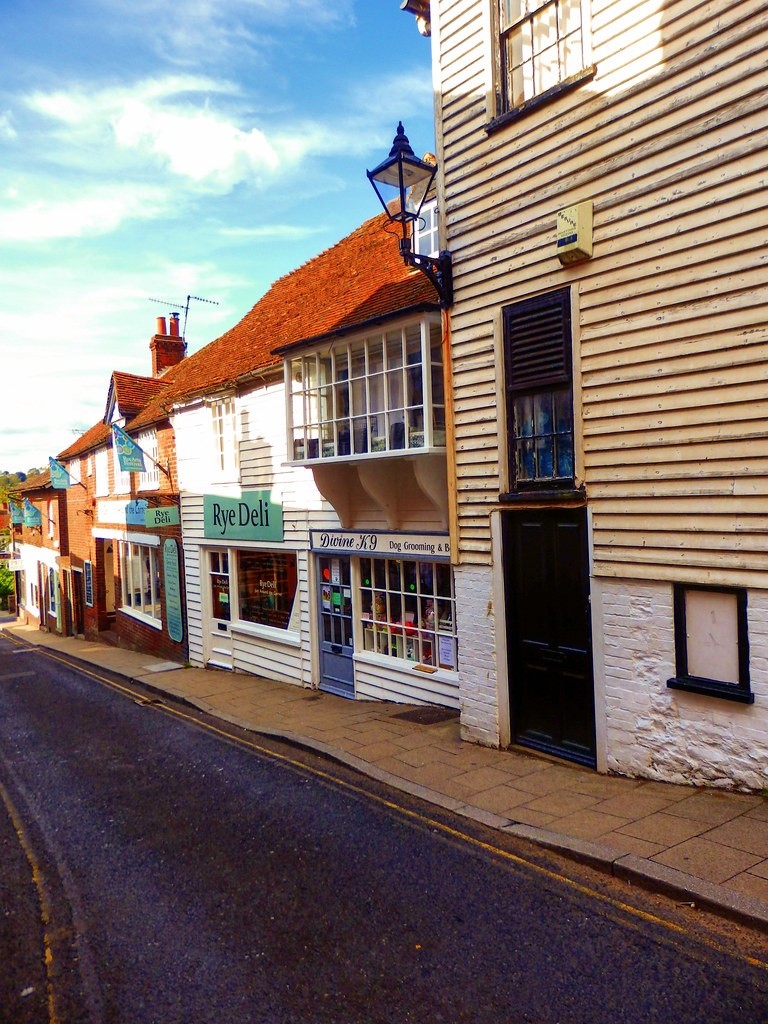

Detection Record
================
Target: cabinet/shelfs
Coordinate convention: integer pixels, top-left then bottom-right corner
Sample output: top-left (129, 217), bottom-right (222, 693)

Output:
top-left (365, 628), bottom-right (435, 666)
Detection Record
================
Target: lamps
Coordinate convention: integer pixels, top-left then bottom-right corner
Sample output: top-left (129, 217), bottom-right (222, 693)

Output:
top-left (365, 121), bottom-right (453, 310)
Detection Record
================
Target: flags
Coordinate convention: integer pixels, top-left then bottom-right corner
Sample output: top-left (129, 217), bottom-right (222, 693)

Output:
top-left (49, 457), bottom-right (72, 489)
top-left (112, 423), bottom-right (145, 473)
top-left (10, 500), bottom-right (42, 528)
